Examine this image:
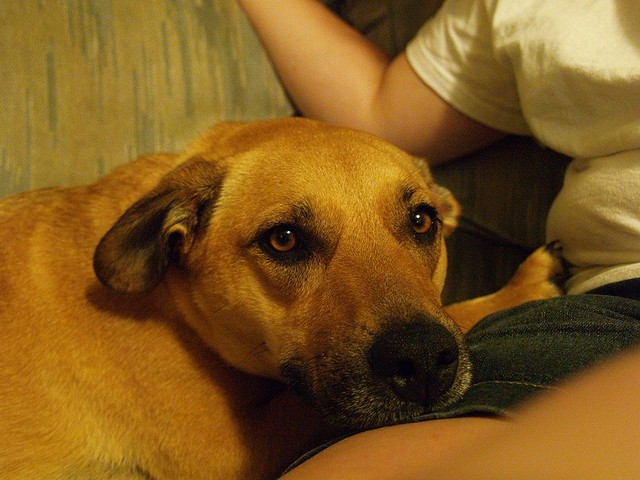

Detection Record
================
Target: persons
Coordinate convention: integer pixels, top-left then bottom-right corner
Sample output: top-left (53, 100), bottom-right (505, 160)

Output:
top-left (234, 0), bottom-right (639, 479)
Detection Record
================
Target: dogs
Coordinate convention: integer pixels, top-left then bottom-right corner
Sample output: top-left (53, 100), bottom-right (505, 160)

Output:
top-left (0, 117), bottom-right (573, 480)
